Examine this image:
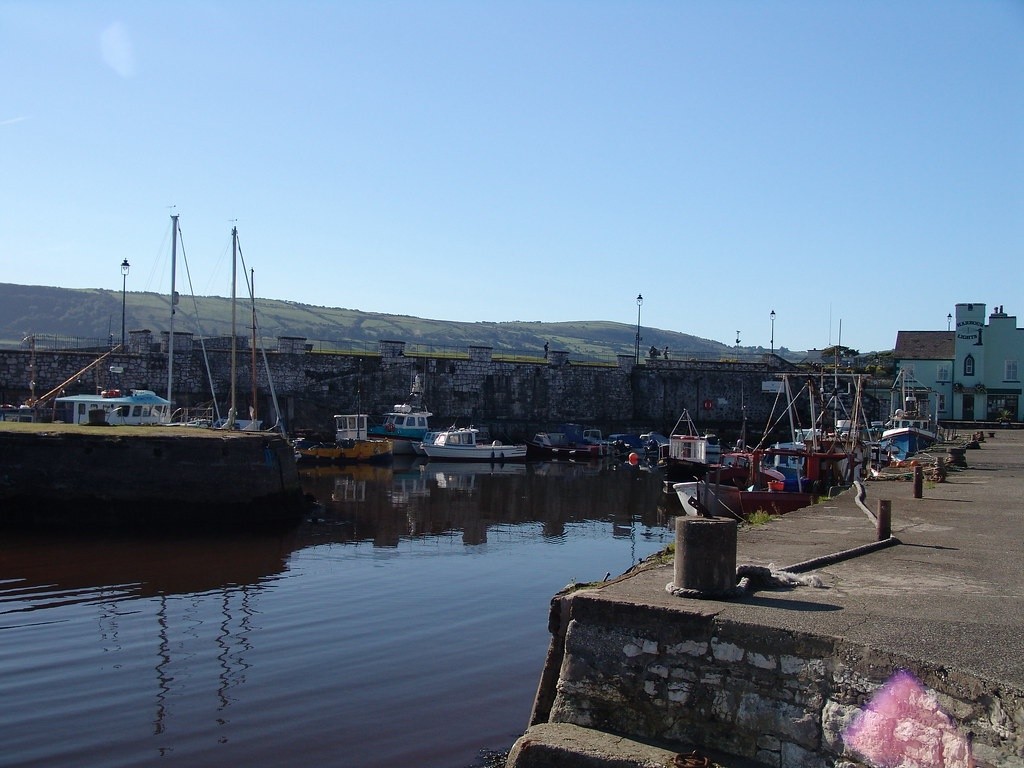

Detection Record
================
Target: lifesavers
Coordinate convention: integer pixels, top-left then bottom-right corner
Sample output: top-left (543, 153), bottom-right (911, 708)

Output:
top-left (386, 423), bottom-right (395, 432)
top-left (375, 447), bottom-right (383, 456)
top-left (704, 400), bottom-right (712, 411)
top-left (101, 389), bottom-right (121, 397)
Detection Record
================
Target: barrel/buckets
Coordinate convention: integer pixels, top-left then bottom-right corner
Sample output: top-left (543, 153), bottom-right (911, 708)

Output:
top-left (767, 480), bottom-right (784, 490)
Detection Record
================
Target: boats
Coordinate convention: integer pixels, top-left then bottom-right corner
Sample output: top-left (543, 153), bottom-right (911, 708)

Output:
top-left (294, 357), bottom-right (394, 468)
top-left (412, 413), bottom-right (528, 460)
top-left (522, 405), bottom-right (723, 476)
top-left (368, 345), bottom-right (434, 440)
top-left (326, 472), bottom-right (432, 507)
top-left (416, 461), bottom-right (525, 493)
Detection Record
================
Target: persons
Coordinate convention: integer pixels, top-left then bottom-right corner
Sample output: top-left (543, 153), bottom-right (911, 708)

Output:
top-left (649, 346), bottom-right (656, 358)
top-left (664, 347), bottom-right (669, 359)
top-left (544, 342), bottom-right (549, 359)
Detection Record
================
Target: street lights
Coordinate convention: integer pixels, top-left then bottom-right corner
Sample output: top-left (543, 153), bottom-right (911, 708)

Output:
top-left (634, 292), bottom-right (644, 367)
top-left (119, 256), bottom-right (131, 356)
top-left (947, 313), bottom-right (952, 331)
top-left (770, 309), bottom-right (776, 353)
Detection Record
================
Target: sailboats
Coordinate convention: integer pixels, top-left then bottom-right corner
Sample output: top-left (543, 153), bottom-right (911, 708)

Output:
top-left (0, 213), bottom-right (294, 446)
top-left (672, 317), bottom-right (948, 526)
